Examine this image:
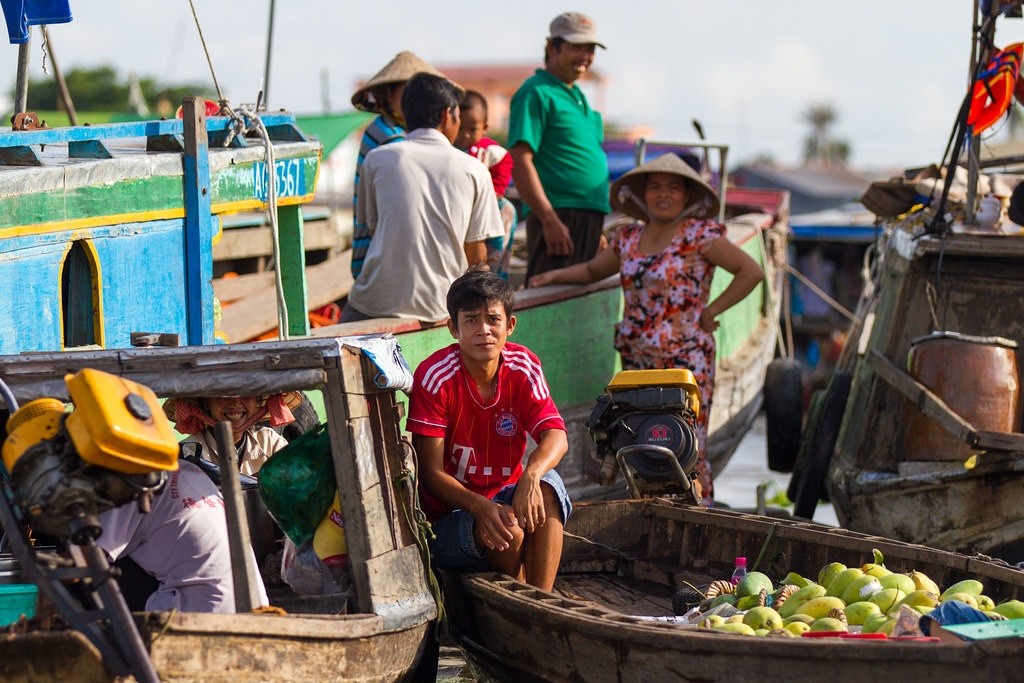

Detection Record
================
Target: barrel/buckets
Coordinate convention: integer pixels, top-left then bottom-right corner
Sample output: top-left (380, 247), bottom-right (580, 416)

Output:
top-left (0, 583), bottom-right (39, 627)
top-left (904, 335), bottom-right (1017, 461)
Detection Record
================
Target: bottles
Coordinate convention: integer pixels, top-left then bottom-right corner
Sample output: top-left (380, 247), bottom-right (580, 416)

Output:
top-left (731, 557), bottom-right (748, 585)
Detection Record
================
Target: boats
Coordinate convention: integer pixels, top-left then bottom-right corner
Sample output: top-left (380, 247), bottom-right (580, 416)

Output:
top-left (0, 1), bottom-right (1024, 682)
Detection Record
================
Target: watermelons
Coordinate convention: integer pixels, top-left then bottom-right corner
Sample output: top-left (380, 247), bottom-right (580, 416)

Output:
top-left (175, 97), bottom-right (222, 117)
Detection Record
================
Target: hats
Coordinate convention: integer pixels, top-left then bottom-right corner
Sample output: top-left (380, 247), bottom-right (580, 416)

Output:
top-left (609, 152), bottom-right (720, 221)
top-left (163, 384), bottom-right (303, 433)
top-left (351, 52), bottom-right (465, 113)
top-left (550, 11), bottom-right (607, 50)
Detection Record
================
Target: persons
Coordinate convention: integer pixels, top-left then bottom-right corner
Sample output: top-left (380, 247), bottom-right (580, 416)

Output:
top-left (404, 270), bottom-right (573, 592)
top-left (529, 151), bottom-right (763, 507)
top-left (508, 12), bottom-right (611, 289)
top-left (79, 388), bottom-right (349, 614)
top-left (337, 51), bottom-right (516, 325)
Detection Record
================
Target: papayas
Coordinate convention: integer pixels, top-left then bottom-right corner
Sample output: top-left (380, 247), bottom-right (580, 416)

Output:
top-left (695, 560), bottom-right (1024, 636)
